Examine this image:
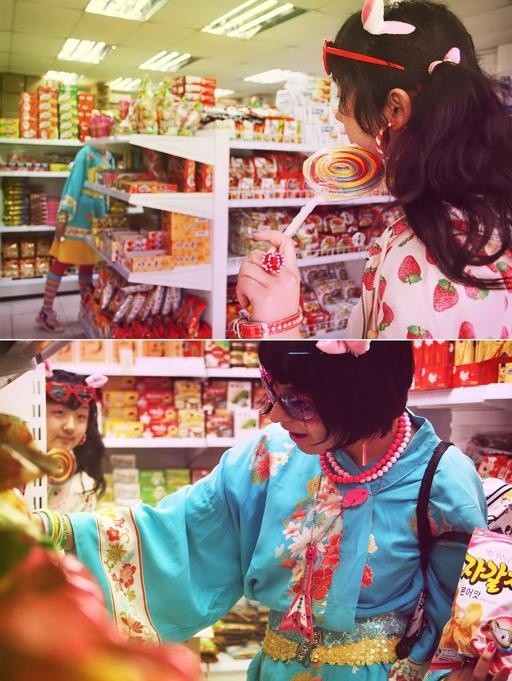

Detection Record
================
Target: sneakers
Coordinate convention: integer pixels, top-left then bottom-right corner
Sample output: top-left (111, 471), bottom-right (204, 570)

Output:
top-left (34, 308), bottom-right (66, 334)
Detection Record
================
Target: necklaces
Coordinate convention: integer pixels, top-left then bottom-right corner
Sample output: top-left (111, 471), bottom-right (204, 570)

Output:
top-left (317, 414), bottom-right (413, 488)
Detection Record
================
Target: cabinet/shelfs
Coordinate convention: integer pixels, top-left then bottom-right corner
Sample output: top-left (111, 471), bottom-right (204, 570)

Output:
top-left (46, 361), bottom-right (291, 676)
top-left (1, 132), bottom-right (401, 338)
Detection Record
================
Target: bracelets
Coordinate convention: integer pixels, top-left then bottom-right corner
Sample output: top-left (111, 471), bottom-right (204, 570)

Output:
top-left (53, 213), bottom-right (67, 224)
top-left (229, 310), bottom-right (305, 340)
top-left (32, 506), bottom-right (69, 551)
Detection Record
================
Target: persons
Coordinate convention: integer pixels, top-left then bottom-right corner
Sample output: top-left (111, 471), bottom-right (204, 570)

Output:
top-left (27, 342), bottom-right (488, 681)
top-left (32, 147), bottom-right (116, 334)
top-left (0, 413), bottom-right (208, 679)
top-left (231, 3), bottom-right (511, 339)
top-left (46, 358), bottom-right (107, 514)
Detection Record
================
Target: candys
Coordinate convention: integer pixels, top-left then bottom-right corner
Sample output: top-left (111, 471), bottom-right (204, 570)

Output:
top-left (268, 146), bottom-right (384, 255)
top-left (45, 447), bottom-right (75, 487)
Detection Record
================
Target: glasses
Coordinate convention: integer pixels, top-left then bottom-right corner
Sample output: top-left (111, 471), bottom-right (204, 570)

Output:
top-left (260, 374), bottom-right (321, 422)
top-left (322, 39), bottom-right (409, 78)
top-left (46, 381), bottom-right (97, 405)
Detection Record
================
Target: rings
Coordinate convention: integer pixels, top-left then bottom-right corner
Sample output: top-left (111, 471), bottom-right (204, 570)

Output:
top-left (259, 251), bottom-right (285, 273)
